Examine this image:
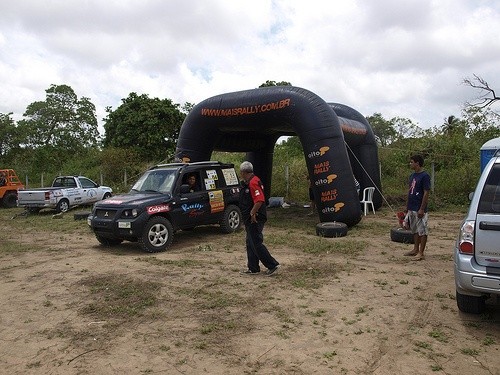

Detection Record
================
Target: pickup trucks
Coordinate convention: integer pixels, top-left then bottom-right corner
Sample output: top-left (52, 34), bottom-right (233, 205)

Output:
top-left (16, 175), bottom-right (113, 215)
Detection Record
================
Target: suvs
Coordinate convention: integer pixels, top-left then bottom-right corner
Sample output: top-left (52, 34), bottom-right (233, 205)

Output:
top-left (456, 148), bottom-right (500, 315)
top-left (86, 160), bottom-right (243, 253)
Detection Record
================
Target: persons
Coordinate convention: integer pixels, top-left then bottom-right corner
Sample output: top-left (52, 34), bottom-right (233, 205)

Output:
top-left (186, 173), bottom-right (200, 193)
top-left (239, 161), bottom-right (280, 278)
top-left (403, 154), bottom-right (431, 261)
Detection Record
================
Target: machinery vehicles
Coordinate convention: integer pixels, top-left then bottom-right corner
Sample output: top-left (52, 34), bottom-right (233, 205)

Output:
top-left (0, 169), bottom-right (25, 208)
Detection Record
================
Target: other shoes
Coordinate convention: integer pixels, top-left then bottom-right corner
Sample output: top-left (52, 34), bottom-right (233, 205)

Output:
top-left (262, 264), bottom-right (282, 276)
top-left (403, 249), bottom-right (419, 256)
top-left (411, 254), bottom-right (426, 261)
top-left (239, 269), bottom-right (261, 275)
